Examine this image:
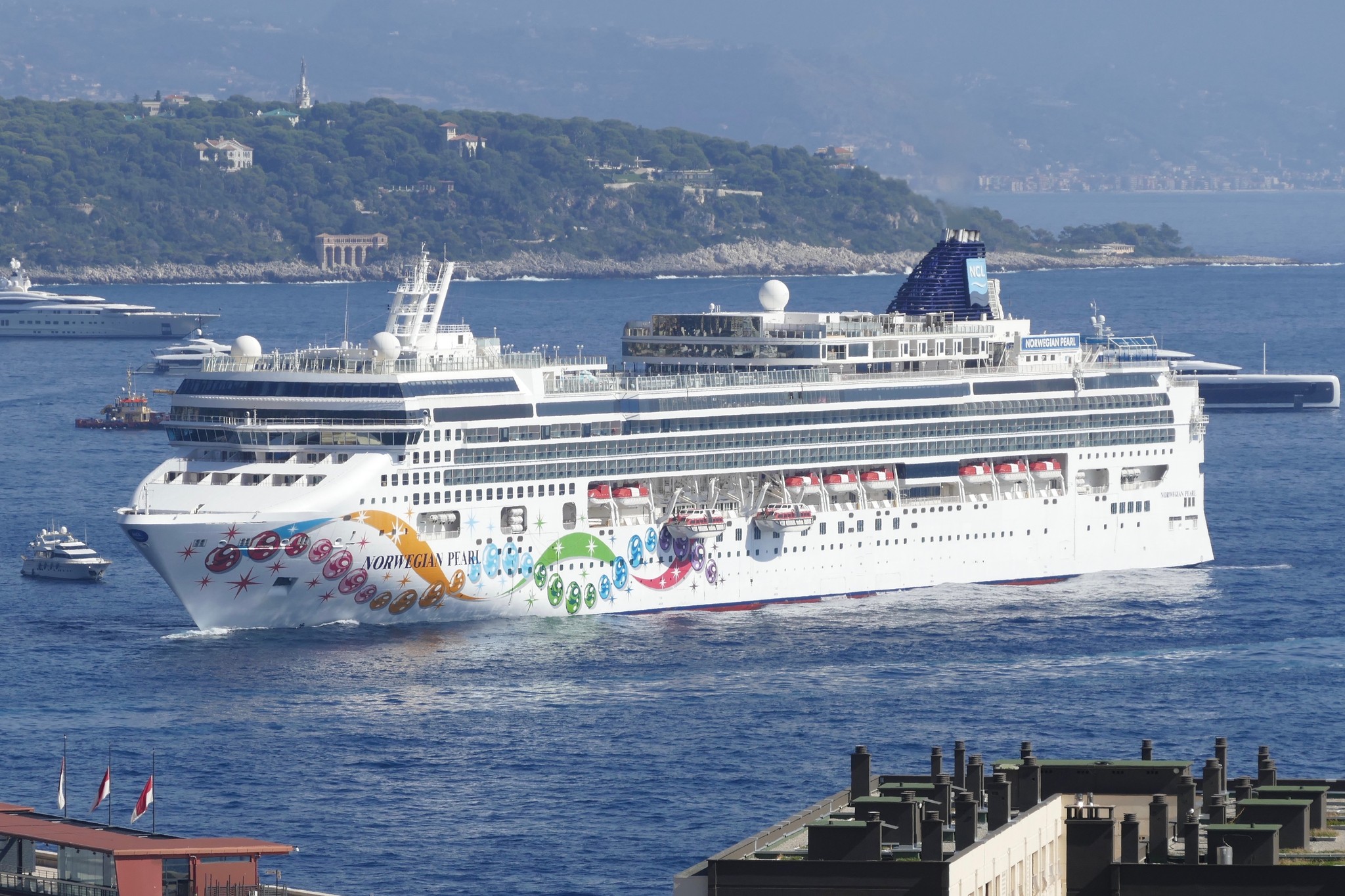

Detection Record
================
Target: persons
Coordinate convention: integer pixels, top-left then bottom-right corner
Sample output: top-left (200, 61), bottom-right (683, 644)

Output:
top-left (320, 364), bottom-right (322, 371)
top-left (335, 367), bottom-right (345, 371)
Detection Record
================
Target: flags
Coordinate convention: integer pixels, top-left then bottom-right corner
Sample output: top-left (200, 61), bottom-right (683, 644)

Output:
top-left (57, 757), bottom-right (65, 810)
top-left (130, 774), bottom-right (154, 825)
top-left (91, 765), bottom-right (110, 812)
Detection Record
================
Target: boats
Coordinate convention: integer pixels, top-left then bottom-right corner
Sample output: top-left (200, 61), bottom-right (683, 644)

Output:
top-left (75, 363), bottom-right (169, 430)
top-left (784, 472), bottom-right (821, 495)
top-left (1029, 458), bottom-right (1062, 480)
top-left (860, 468), bottom-right (895, 490)
top-left (959, 461), bottom-right (992, 484)
top-left (823, 470), bottom-right (858, 492)
top-left (1104, 348), bottom-right (1340, 410)
top-left (587, 484), bottom-right (611, 504)
top-left (754, 503), bottom-right (814, 532)
top-left (0, 257), bottom-right (222, 339)
top-left (20, 518), bottom-right (113, 581)
top-left (612, 483), bottom-right (651, 507)
top-left (132, 316), bottom-right (231, 375)
top-left (665, 508), bottom-right (726, 539)
top-left (994, 459), bottom-right (1027, 482)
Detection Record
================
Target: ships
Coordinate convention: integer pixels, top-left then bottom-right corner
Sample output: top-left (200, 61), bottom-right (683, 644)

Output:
top-left (115, 225), bottom-right (1218, 638)
